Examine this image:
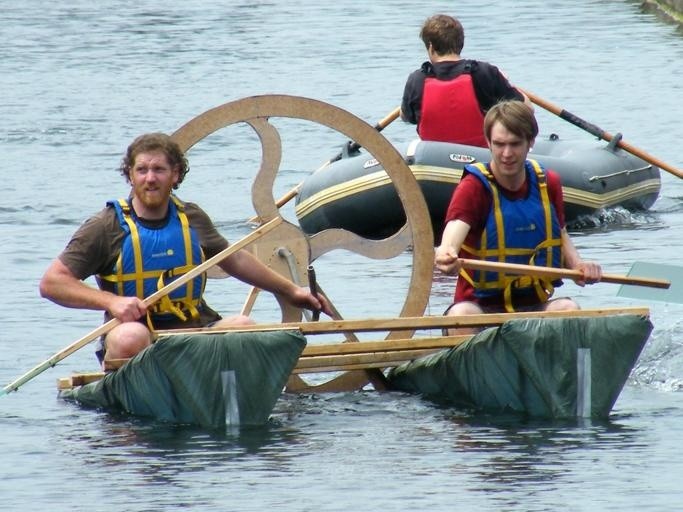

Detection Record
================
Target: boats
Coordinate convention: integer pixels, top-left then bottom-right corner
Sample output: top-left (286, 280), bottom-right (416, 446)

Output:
top-left (54, 327), bottom-right (311, 436)
top-left (292, 136), bottom-right (664, 236)
top-left (378, 309), bottom-right (646, 432)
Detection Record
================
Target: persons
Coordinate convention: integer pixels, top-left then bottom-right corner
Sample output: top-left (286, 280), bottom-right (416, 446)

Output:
top-left (39, 132), bottom-right (335, 370)
top-left (399, 14), bottom-right (536, 148)
top-left (430, 101), bottom-right (603, 337)
